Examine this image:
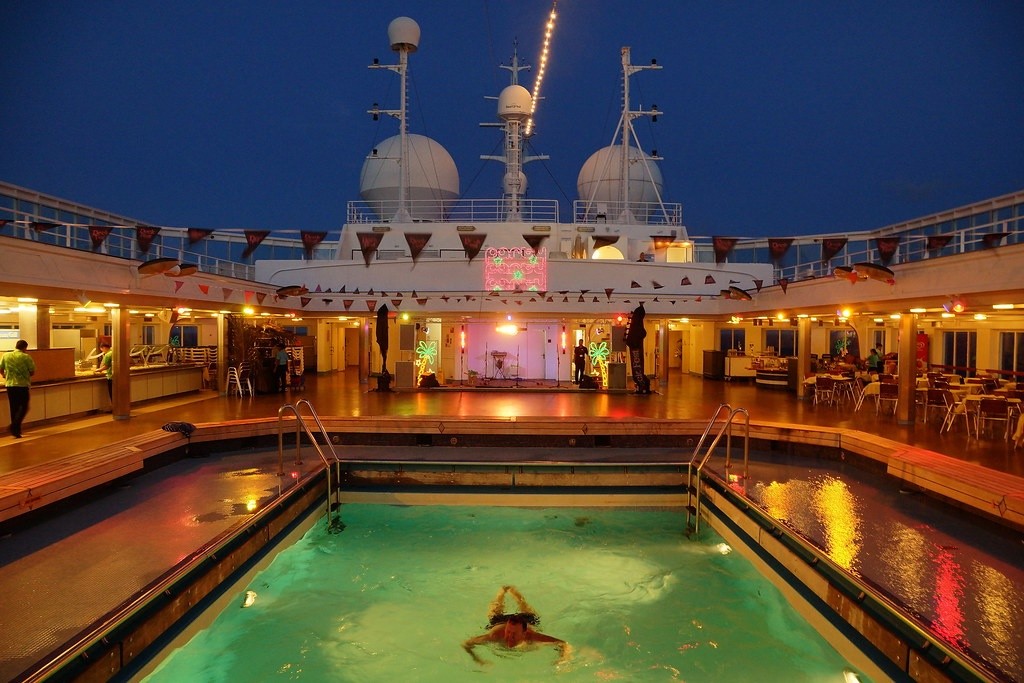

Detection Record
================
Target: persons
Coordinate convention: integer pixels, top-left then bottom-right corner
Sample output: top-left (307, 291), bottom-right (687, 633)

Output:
top-left (94, 342), bottom-right (114, 414)
top-left (863, 342), bottom-right (884, 374)
top-left (462, 585), bottom-right (568, 669)
top-left (0, 340), bottom-right (37, 439)
top-left (269, 337), bottom-right (290, 395)
top-left (573, 339), bottom-right (590, 384)
top-left (637, 252), bottom-right (649, 262)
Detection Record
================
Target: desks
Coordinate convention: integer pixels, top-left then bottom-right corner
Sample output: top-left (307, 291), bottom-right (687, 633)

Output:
top-left (950, 382), bottom-right (986, 395)
top-left (802, 375), bottom-right (853, 407)
top-left (0, 364), bottom-right (207, 433)
top-left (855, 382), bottom-right (880, 412)
top-left (703, 350), bottom-right (724, 380)
top-left (396, 361), bottom-right (415, 386)
top-left (916, 388), bottom-right (967, 421)
top-left (854, 370), bottom-right (867, 377)
top-left (916, 378), bottom-right (928, 387)
top-left (993, 388), bottom-right (1024, 395)
top-left (756, 367), bottom-right (788, 391)
top-left (872, 374), bottom-right (897, 404)
top-left (608, 362), bottom-right (626, 390)
top-left (1004, 382), bottom-right (1024, 389)
top-left (964, 378), bottom-right (1009, 384)
top-left (724, 357), bottom-right (757, 381)
top-left (923, 373), bottom-right (962, 384)
top-left (491, 353), bottom-right (508, 381)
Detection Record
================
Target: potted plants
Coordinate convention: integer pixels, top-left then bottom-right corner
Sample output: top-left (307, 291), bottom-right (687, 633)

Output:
top-left (376, 303), bottom-right (393, 391)
top-left (464, 369), bottom-right (482, 386)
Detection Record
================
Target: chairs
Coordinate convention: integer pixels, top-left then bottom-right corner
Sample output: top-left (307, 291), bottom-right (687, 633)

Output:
top-left (290, 369), bottom-right (306, 392)
top-left (225, 361), bottom-right (253, 397)
top-left (172, 345), bottom-right (217, 389)
top-left (811, 353), bottom-right (1024, 448)
top-left (286, 346), bottom-right (304, 386)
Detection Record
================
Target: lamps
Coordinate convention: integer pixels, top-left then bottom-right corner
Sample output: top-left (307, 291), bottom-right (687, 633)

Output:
top-left (758, 319), bottom-right (762, 325)
top-left (819, 319), bottom-right (824, 326)
top-left (275, 286), bottom-right (301, 304)
top-left (496, 322), bottom-right (528, 335)
top-left (752, 319), bottom-right (757, 326)
top-left (129, 258), bottom-right (199, 288)
top-left (769, 319), bottom-right (773, 326)
top-left (790, 317), bottom-right (798, 326)
top-left (77, 290), bottom-right (92, 307)
top-left (833, 319), bottom-right (840, 326)
top-left (833, 266), bottom-right (867, 283)
top-left (421, 327), bottom-right (429, 334)
top-left (853, 262), bottom-right (895, 286)
top-left (943, 294), bottom-right (964, 313)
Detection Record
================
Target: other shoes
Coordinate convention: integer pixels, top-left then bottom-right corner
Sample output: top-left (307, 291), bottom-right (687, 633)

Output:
top-left (575, 379), bottom-right (578, 383)
top-left (8, 424), bottom-right (22, 438)
